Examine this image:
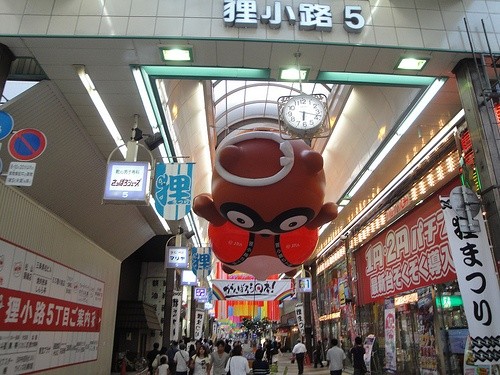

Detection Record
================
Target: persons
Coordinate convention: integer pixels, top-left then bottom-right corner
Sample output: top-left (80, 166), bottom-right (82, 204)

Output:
top-left (148, 336), bottom-right (367, 375)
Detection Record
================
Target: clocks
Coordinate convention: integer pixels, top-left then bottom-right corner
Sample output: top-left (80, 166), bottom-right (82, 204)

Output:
top-left (278, 93), bottom-right (332, 140)
